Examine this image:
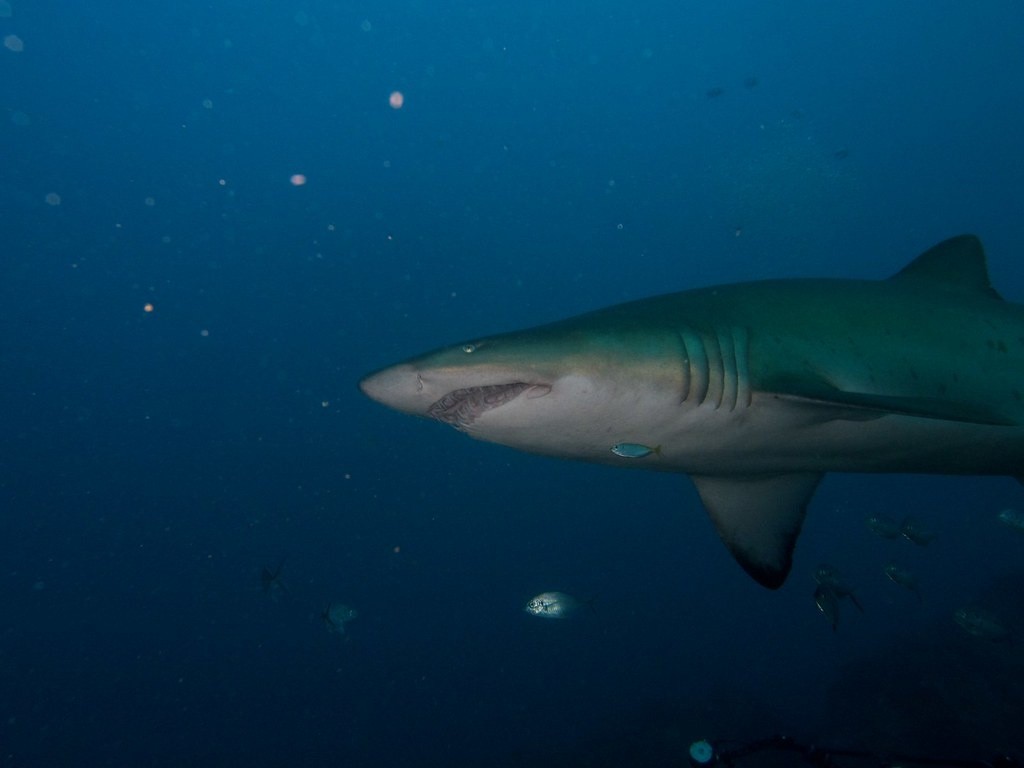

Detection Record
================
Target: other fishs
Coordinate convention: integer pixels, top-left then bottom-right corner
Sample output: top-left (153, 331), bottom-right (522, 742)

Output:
top-left (321, 599), bottom-right (352, 633)
top-left (522, 591), bottom-right (597, 619)
top-left (815, 509), bottom-right (1024, 657)
